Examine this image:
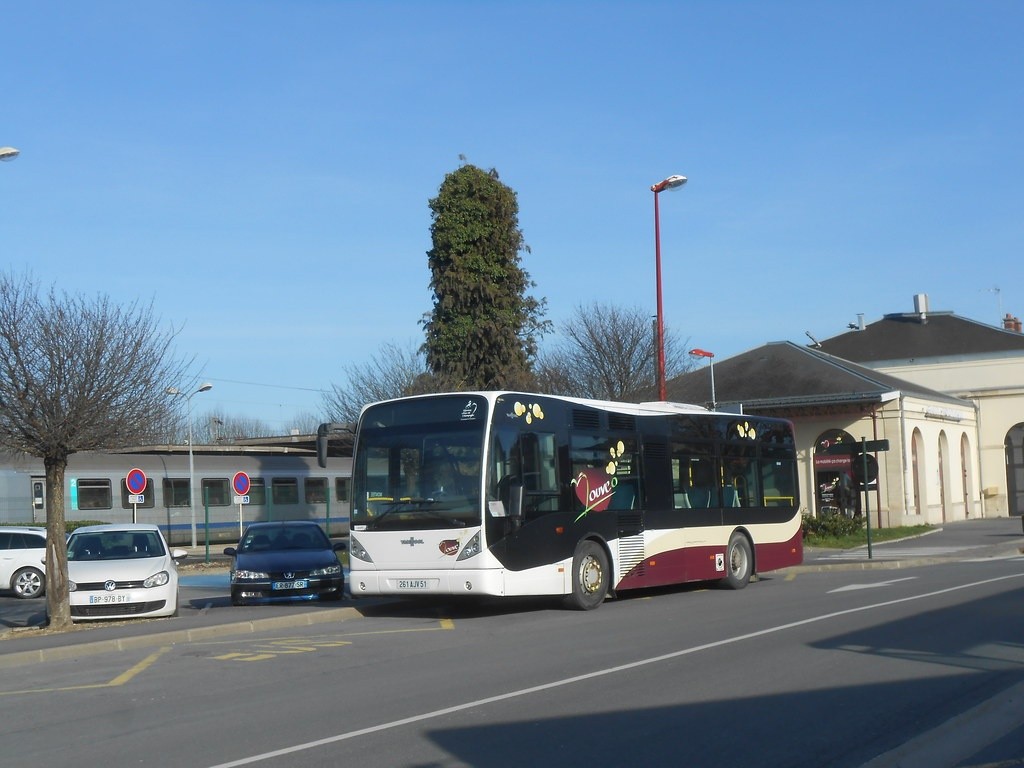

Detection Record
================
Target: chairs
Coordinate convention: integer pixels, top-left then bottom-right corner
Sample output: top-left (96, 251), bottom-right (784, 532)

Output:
top-left (609, 485), bottom-right (743, 509)
top-left (253, 534), bottom-right (271, 551)
top-left (293, 534), bottom-right (311, 547)
top-left (76, 537), bottom-right (105, 558)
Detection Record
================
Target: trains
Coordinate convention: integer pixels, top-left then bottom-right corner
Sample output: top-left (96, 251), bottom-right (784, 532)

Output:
top-left (0, 444), bottom-right (501, 547)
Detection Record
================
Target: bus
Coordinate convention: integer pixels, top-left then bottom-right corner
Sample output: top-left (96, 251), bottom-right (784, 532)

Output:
top-left (317, 389), bottom-right (804, 611)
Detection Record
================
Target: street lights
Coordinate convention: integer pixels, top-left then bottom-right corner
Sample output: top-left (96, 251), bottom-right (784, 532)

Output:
top-left (163, 384), bottom-right (213, 549)
top-left (651, 175), bottom-right (687, 401)
top-left (689, 349), bottom-right (718, 412)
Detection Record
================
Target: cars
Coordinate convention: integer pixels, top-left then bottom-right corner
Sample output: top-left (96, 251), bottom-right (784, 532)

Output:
top-left (41, 522), bottom-right (189, 627)
top-left (0, 525), bottom-right (71, 598)
top-left (222, 521), bottom-right (346, 606)
